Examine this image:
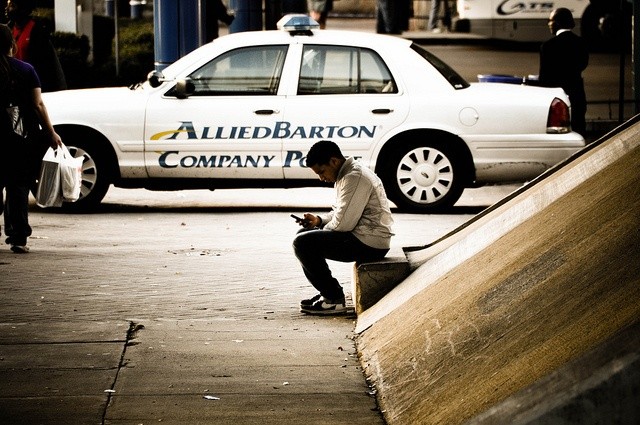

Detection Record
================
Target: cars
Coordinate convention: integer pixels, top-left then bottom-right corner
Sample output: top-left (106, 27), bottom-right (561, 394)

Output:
top-left (30, 12), bottom-right (585, 214)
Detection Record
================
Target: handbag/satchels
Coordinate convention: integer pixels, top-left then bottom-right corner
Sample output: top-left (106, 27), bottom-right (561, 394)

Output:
top-left (2, 102), bottom-right (26, 139)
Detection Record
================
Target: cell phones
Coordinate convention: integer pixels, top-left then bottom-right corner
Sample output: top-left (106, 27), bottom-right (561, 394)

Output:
top-left (291, 214), bottom-right (304, 221)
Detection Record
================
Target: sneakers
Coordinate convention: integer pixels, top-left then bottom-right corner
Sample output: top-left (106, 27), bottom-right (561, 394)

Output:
top-left (301, 289), bottom-right (344, 305)
top-left (301, 296), bottom-right (347, 314)
top-left (10, 244), bottom-right (29, 254)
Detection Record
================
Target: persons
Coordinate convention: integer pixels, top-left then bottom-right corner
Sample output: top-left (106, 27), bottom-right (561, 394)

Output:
top-left (201, 0), bottom-right (237, 89)
top-left (376, 0), bottom-right (415, 36)
top-left (0, 24), bottom-right (63, 254)
top-left (292, 141), bottom-right (395, 315)
top-left (539, 8), bottom-right (590, 139)
top-left (307, 0), bottom-right (334, 29)
